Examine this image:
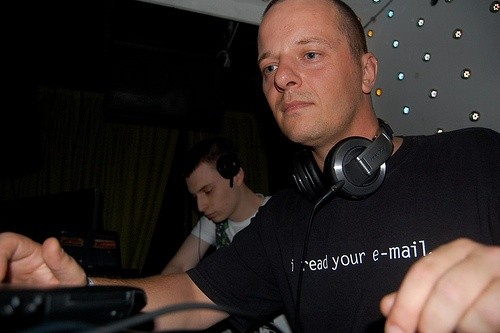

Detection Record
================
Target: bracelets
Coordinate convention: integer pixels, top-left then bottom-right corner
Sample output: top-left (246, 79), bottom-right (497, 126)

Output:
top-left (87, 277), bottom-right (95, 286)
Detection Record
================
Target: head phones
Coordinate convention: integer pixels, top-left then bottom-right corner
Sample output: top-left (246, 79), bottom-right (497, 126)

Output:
top-left (287, 116), bottom-right (394, 199)
top-left (215, 150), bottom-right (241, 179)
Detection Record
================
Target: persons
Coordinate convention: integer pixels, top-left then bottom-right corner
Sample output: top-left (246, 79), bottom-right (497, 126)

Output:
top-left (161, 138), bottom-right (292, 333)
top-left (0, 0), bottom-right (500, 333)
